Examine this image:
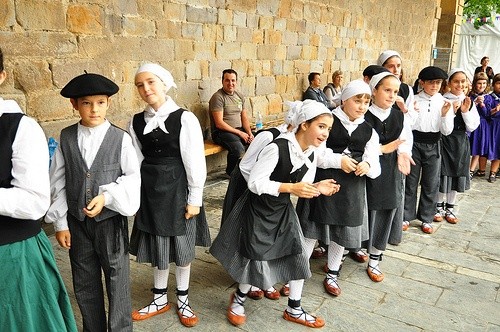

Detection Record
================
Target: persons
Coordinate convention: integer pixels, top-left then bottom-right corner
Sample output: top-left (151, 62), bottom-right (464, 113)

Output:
top-left (0, 47), bottom-right (79, 332)
top-left (44, 74), bottom-right (142, 332)
top-left (312, 50), bottom-right (419, 261)
top-left (208, 69), bottom-right (257, 177)
top-left (402, 56), bottom-right (500, 233)
top-left (302, 72), bottom-right (335, 111)
top-left (207, 98), bottom-right (341, 329)
top-left (127, 64), bottom-right (211, 327)
top-left (323, 70), bottom-right (345, 106)
top-left (294, 78), bottom-right (383, 297)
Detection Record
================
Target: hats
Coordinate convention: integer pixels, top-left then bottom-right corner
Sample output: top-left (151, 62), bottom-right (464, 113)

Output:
top-left (60, 70), bottom-right (119, 99)
top-left (283, 99), bottom-right (303, 124)
top-left (369, 72), bottom-right (400, 92)
top-left (363, 65), bottom-right (391, 79)
top-left (447, 68), bottom-right (467, 81)
top-left (377, 50), bottom-right (402, 67)
top-left (341, 79), bottom-right (371, 102)
top-left (418, 67), bottom-right (448, 81)
top-left (294, 99), bottom-right (332, 126)
top-left (135, 63), bottom-right (178, 94)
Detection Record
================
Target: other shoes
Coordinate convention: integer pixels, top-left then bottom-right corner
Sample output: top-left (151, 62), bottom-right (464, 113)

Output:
top-left (283, 298), bottom-right (325, 328)
top-left (324, 260), bottom-right (341, 296)
top-left (422, 221), bottom-right (433, 234)
top-left (401, 220), bottom-right (410, 231)
top-left (367, 253), bottom-right (384, 282)
top-left (349, 249), bottom-right (368, 263)
top-left (489, 167), bottom-right (500, 182)
top-left (468, 169), bottom-right (485, 180)
top-left (175, 290), bottom-right (198, 327)
top-left (246, 282), bottom-right (292, 300)
top-left (227, 287), bottom-right (248, 325)
top-left (444, 203), bottom-right (458, 224)
top-left (310, 246), bottom-right (327, 259)
top-left (131, 291), bottom-right (169, 321)
top-left (433, 202), bottom-right (444, 222)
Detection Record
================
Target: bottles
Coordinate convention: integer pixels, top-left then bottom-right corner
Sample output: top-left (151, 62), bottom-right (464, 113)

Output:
top-left (47, 137), bottom-right (59, 165)
top-left (255, 111), bottom-right (262, 132)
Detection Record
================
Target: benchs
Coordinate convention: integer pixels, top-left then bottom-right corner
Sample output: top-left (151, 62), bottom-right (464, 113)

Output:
top-left (203, 116), bottom-right (285, 157)
